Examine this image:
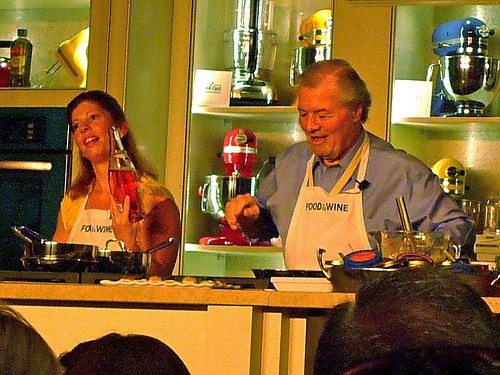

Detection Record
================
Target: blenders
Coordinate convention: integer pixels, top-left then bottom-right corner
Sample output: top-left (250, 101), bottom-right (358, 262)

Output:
top-left (223, 0), bottom-right (282, 107)
top-left (426, 17), bottom-right (500, 118)
top-left (198, 127), bottom-right (277, 246)
top-left (288, 9), bottom-right (334, 87)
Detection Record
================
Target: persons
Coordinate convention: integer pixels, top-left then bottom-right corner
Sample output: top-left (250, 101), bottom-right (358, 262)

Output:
top-left (0, 261), bottom-right (500, 375)
top-left (51, 89), bottom-right (181, 280)
top-left (223, 58), bottom-right (477, 272)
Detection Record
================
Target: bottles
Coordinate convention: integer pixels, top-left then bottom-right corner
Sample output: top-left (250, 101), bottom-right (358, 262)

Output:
top-left (7, 28), bottom-right (32, 87)
top-left (106, 126), bottom-right (147, 223)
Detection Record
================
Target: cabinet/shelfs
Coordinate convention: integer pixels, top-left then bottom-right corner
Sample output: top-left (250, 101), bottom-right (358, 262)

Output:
top-left (0, 0), bottom-right (131, 265)
top-left (164, 0), bottom-right (500, 277)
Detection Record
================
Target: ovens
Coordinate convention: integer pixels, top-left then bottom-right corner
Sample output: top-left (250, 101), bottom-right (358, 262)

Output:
top-left (0, 107), bottom-right (75, 269)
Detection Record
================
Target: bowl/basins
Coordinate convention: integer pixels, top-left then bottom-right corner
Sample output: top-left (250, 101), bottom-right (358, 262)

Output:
top-left (380, 229), bottom-right (449, 265)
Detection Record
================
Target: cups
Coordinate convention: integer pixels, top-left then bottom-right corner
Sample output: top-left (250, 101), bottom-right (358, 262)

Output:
top-left (459, 196), bottom-right (500, 233)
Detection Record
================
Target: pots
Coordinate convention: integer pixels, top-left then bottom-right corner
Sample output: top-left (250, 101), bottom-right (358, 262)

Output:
top-left (96, 236), bottom-right (176, 274)
top-left (17, 225), bottom-right (97, 273)
top-left (12, 226), bottom-right (35, 271)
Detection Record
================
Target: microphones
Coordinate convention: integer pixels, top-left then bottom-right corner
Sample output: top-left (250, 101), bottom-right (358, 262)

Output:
top-left (358, 180), bottom-right (370, 190)
